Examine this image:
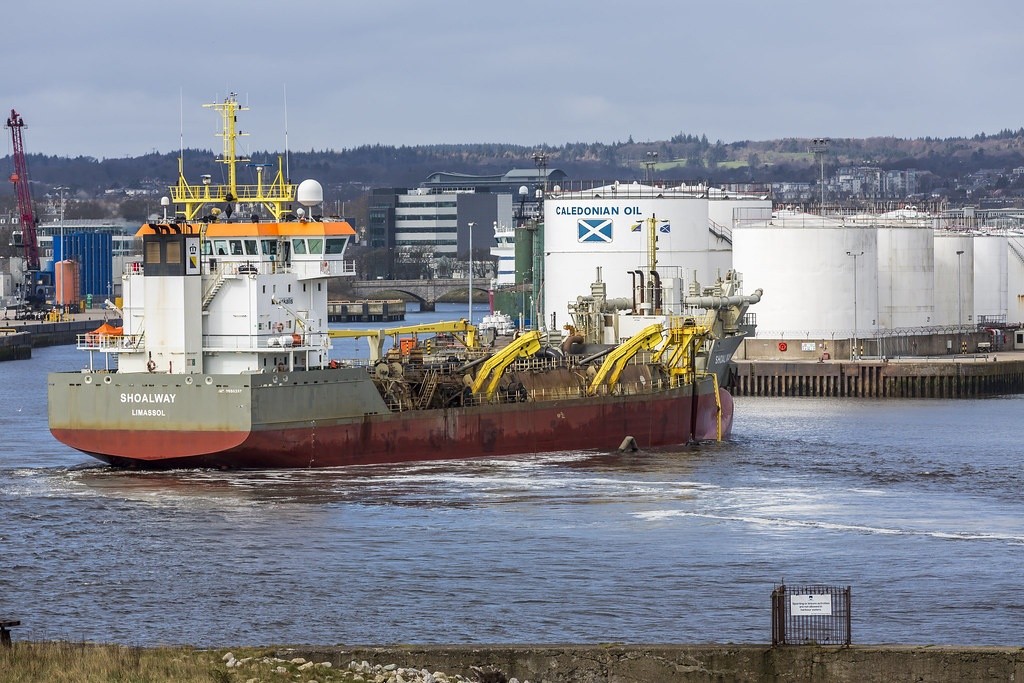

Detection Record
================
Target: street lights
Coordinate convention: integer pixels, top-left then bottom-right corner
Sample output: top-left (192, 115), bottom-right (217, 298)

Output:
top-left (468, 222), bottom-right (478, 326)
top-left (845, 250), bottom-right (865, 359)
top-left (643, 149), bottom-right (658, 181)
top-left (955, 248), bottom-right (965, 353)
top-left (810, 136), bottom-right (830, 228)
top-left (531, 149), bottom-right (547, 221)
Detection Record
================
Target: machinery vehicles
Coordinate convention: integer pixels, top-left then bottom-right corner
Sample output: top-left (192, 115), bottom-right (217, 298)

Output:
top-left (4, 108), bottom-right (57, 322)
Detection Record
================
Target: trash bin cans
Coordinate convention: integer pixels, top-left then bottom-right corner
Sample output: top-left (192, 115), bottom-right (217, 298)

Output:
top-left (115, 297), bottom-right (123, 310)
top-left (822, 352), bottom-right (829, 359)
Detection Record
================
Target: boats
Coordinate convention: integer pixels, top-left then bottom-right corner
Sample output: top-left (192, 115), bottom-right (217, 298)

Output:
top-left (45, 80), bottom-right (766, 470)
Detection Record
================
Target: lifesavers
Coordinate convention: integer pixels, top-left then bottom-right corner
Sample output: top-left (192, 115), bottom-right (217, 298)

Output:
top-left (278, 323), bottom-right (284, 332)
top-left (779, 343), bottom-right (787, 350)
top-left (148, 361), bottom-right (156, 370)
top-left (278, 362), bottom-right (284, 371)
